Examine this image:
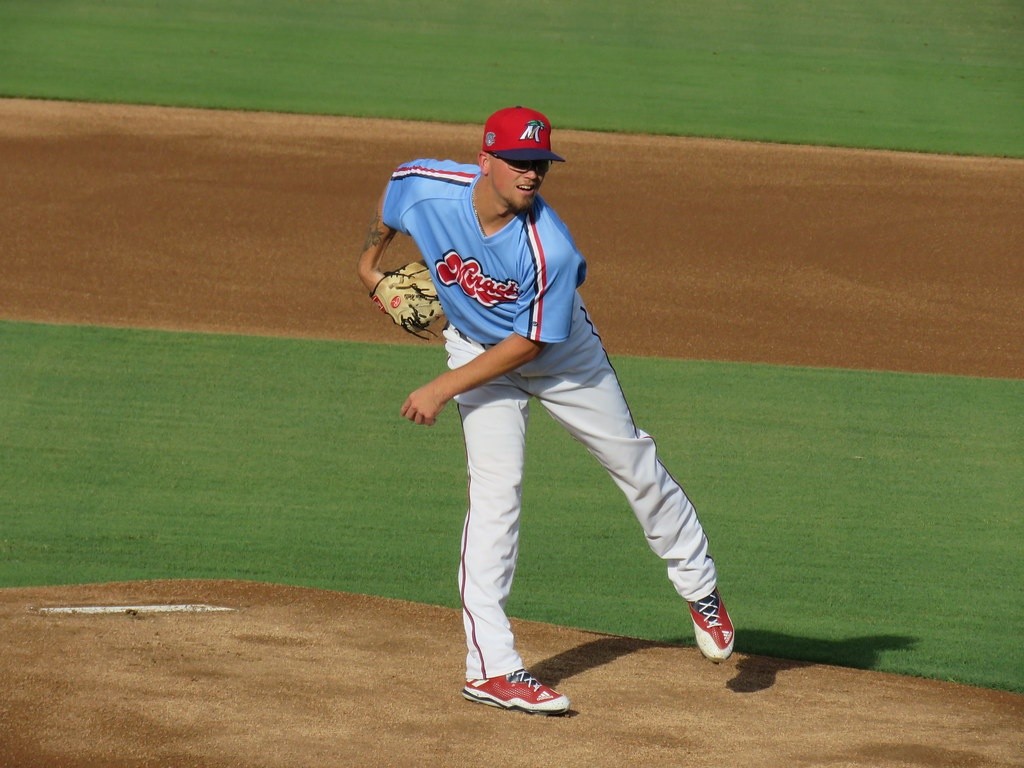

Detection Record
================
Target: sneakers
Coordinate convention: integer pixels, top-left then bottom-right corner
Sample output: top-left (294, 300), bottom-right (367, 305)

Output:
top-left (688, 587), bottom-right (735, 662)
top-left (461, 670), bottom-right (570, 716)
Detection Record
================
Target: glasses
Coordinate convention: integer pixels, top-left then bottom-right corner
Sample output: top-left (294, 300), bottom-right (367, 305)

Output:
top-left (492, 153), bottom-right (552, 174)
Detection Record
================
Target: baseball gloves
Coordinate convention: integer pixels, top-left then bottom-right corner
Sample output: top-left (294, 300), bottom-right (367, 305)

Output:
top-left (367, 260), bottom-right (444, 342)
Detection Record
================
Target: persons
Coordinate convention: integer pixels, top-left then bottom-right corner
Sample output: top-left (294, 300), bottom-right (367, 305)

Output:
top-left (355, 105), bottom-right (735, 713)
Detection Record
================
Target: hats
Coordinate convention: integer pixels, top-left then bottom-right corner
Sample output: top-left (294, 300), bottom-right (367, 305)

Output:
top-left (483, 106), bottom-right (566, 162)
